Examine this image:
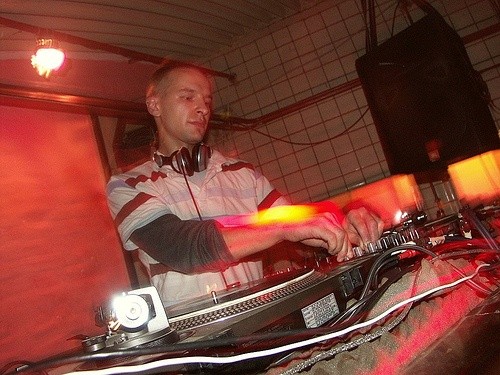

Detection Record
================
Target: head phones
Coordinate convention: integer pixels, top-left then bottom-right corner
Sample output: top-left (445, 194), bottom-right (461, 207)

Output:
top-left (150, 134), bottom-right (213, 176)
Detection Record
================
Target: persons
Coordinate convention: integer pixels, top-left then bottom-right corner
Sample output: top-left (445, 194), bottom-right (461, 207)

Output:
top-left (105, 62), bottom-right (385, 309)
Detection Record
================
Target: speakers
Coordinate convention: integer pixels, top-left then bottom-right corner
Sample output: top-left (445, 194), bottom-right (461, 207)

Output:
top-left (353, 11), bottom-right (500, 185)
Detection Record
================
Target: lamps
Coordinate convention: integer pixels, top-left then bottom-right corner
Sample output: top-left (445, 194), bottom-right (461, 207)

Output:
top-left (34, 26), bottom-right (66, 72)
